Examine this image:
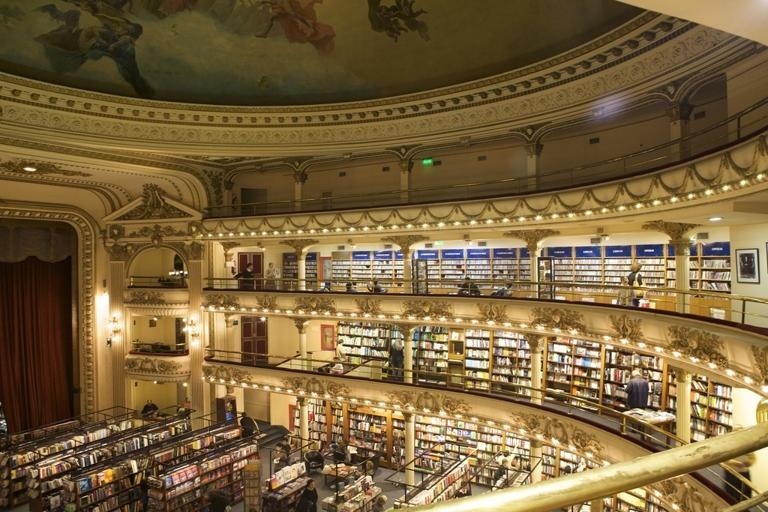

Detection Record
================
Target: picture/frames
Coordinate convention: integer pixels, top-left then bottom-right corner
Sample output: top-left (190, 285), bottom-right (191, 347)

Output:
top-left (735, 248), bottom-right (759, 284)
top-left (766, 242), bottom-right (768, 272)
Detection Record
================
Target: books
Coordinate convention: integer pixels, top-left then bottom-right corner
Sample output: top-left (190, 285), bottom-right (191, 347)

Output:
top-left (2, 260), bottom-right (732, 512)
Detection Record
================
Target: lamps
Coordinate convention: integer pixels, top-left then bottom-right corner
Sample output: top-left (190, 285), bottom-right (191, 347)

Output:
top-left (187, 319), bottom-right (200, 339)
top-left (106, 316), bottom-right (122, 333)
